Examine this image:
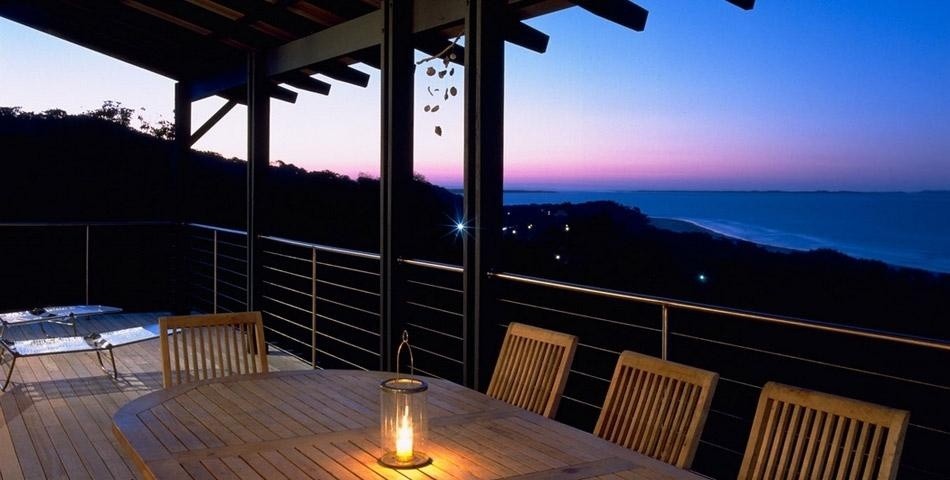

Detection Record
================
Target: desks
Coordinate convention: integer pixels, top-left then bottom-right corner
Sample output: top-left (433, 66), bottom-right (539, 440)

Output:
top-left (111, 370), bottom-right (711, 480)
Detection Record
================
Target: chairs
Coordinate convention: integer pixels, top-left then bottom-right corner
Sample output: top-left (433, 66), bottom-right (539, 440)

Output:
top-left (157, 311), bottom-right (269, 390)
top-left (0, 305), bottom-right (182, 392)
top-left (486, 322), bottom-right (578, 419)
top-left (735, 381), bottom-right (911, 478)
top-left (592, 350), bottom-right (721, 471)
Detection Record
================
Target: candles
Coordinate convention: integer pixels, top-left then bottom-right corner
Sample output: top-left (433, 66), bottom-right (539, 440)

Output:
top-left (396, 415), bottom-right (414, 461)
